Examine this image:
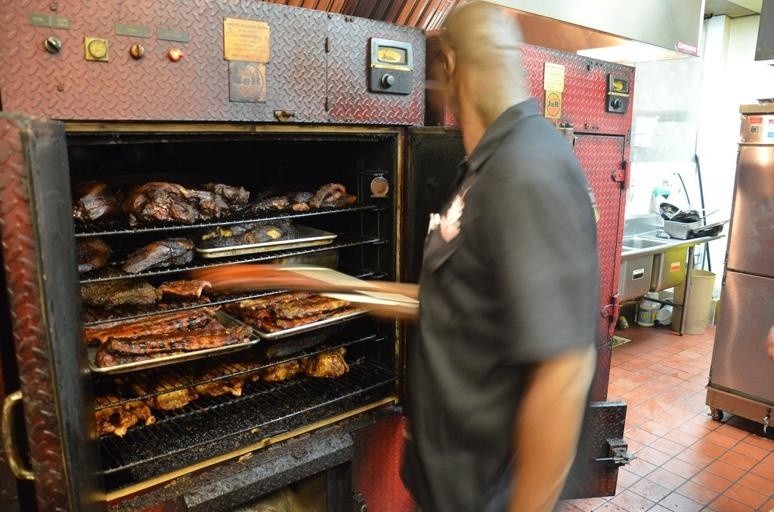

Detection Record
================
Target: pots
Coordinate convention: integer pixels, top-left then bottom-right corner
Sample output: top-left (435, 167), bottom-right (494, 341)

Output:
top-left (692, 219), bottom-right (730, 238)
top-left (658, 202), bottom-right (719, 223)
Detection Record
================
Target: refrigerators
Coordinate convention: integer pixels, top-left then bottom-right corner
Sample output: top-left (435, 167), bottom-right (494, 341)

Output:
top-left (704, 102), bottom-right (774, 438)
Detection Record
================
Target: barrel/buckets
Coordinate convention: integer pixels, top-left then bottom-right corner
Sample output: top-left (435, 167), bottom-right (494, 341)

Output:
top-left (659, 303), bottom-right (675, 325)
top-left (683, 268), bottom-right (718, 335)
top-left (637, 302), bottom-right (662, 327)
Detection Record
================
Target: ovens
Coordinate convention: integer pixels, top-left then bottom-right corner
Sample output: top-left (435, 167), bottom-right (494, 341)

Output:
top-left (0, 1), bottom-right (467, 512)
top-left (426, 30), bottom-right (639, 501)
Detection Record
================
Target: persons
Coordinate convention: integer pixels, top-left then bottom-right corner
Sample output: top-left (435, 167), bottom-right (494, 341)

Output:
top-left (398, 0), bottom-right (600, 512)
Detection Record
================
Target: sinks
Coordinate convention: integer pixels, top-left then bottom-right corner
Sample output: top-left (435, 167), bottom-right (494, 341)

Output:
top-left (621, 246), bottom-right (632, 254)
top-left (623, 236), bottom-right (670, 250)
